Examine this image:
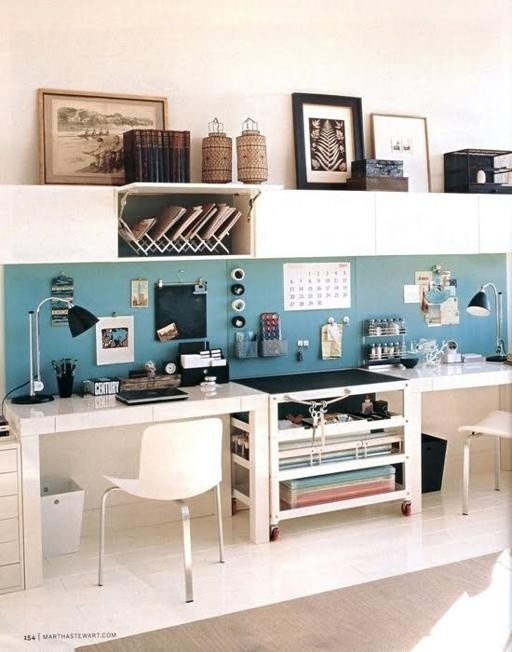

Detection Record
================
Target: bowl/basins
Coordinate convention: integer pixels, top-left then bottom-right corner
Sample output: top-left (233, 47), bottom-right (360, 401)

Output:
top-left (400, 356), bottom-right (419, 368)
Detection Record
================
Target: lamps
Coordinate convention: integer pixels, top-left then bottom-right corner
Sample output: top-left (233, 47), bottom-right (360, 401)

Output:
top-left (11, 297), bottom-right (99, 403)
top-left (466, 282), bottom-right (507, 362)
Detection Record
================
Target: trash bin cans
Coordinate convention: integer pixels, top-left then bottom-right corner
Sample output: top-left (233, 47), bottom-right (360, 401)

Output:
top-left (422, 433), bottom-right (447, 493)
top-left (40, 476), bottom-right (84, 557)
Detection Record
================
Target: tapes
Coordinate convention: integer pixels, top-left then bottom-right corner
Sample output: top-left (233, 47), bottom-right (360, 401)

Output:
top-left (232, 316), bottom-right (245, 328)
top-left (232, 299), bottom-right (245, 312)
top-left (231, 284), bottom-right (245, 296)
top-left (232, 268), bottom-right (245, 281)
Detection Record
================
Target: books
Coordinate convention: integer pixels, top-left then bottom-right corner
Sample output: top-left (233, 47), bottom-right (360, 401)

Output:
top-left (123, 129), bottom-right (190, 183)
top-left (130, 202), bottom-right (243, 241)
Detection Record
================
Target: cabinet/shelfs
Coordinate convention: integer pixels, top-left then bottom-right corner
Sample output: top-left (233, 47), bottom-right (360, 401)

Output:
top-left (0, 447), bottom-right (24, 595)
top-left (279, 414), bottom-right (406, 523)
top-left (366, 322), bottom-right (405, 360)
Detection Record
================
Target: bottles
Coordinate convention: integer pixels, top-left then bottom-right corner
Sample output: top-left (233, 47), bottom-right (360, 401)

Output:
top-left (367, 341), bottom-right (406, 360)
top-left (367, 316), bottom-right (405, 335)
top-left (361, 398), bottom-right (374, 415)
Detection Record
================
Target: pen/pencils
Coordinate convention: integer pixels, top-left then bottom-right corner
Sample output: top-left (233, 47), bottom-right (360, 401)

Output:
top-left (51, 358), bottom-right (77, 376)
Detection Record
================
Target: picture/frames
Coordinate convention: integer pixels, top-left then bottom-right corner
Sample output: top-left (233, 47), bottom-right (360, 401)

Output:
top-left (37, 88), bottom-right (168, 185)
top-left (370, 113), bottom-right (431, 193)
top-left (291, 93), bottom-right (364, 189)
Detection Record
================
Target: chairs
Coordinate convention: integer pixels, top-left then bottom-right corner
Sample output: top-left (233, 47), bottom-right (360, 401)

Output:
top-left (458, 410), bottom-right (512, 516)
top-left (98, 418), bottom-right (225, 603)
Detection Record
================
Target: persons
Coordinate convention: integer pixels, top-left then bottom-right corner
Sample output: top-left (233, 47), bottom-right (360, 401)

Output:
top-left (82, 128), bottom-right (123, 170)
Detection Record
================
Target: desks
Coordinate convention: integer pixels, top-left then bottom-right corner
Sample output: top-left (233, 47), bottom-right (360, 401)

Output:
top-left (3, 381), bottom-right (269, 590)
top-left (368, 358), bottom-right (512, 513)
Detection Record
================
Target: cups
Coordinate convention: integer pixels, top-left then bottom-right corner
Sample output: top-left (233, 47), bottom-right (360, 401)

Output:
top-left (55, 374), bottom-right (75, 399)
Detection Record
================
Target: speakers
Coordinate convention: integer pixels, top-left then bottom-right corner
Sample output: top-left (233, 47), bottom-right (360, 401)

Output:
top-left (392, 433), bottom-right (447, 494)
